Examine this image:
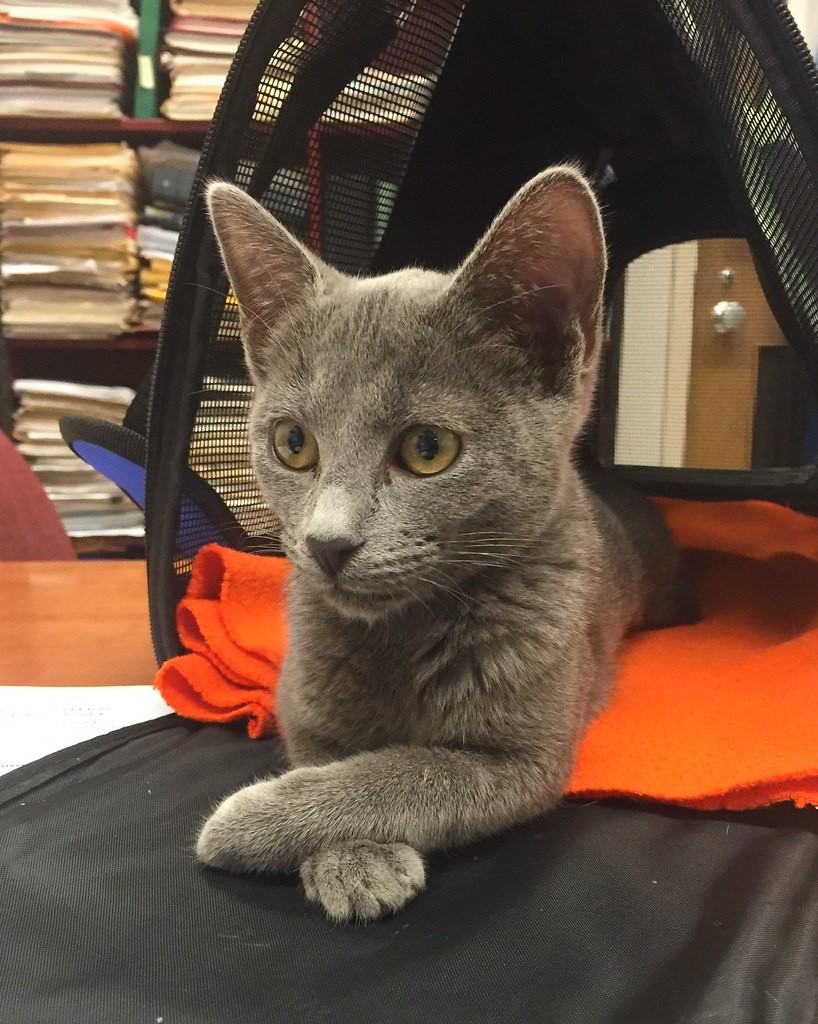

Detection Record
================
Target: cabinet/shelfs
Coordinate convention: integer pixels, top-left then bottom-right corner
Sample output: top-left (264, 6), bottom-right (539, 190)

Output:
top-left (0, 114), bottom-right (418, 552)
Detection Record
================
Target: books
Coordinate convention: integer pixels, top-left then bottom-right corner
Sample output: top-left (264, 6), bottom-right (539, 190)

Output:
top-left (1, 140), bottom-right (203, 339)
top-left (9, 378), bottom-right (147, 536)
top-left (160, 0), bottom-right (259, 120)
top-left (0, 0), bottom-right (140, 120)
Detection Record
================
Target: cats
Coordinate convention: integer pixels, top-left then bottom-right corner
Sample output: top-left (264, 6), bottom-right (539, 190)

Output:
top-left (198, 164), bottom-right (695, 924)
